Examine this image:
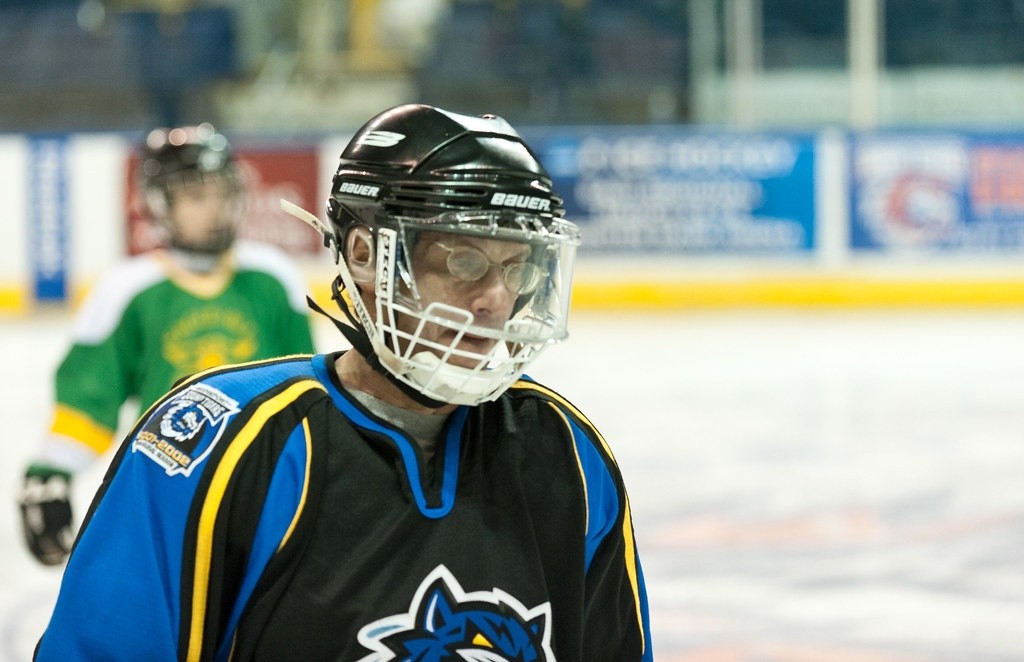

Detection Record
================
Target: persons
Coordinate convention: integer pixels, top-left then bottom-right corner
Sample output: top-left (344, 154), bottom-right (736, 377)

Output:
top-left (12, 117), bottom-right (332, 569)
top-left (31, 107), bottom-right (653, 662)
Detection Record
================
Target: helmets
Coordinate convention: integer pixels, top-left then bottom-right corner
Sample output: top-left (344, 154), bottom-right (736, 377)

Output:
top-left (142, 130), bottom-right (245, 228)
top-left (325, 102), bottom-right (564, 320)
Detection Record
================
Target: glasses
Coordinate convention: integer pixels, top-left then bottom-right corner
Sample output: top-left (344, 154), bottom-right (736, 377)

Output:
top-left (430, 240), bottom-right (554, 297)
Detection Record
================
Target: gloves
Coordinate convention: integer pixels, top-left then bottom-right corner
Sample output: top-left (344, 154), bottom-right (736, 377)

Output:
top-left (20, 463), bottom-right (77, 569)
